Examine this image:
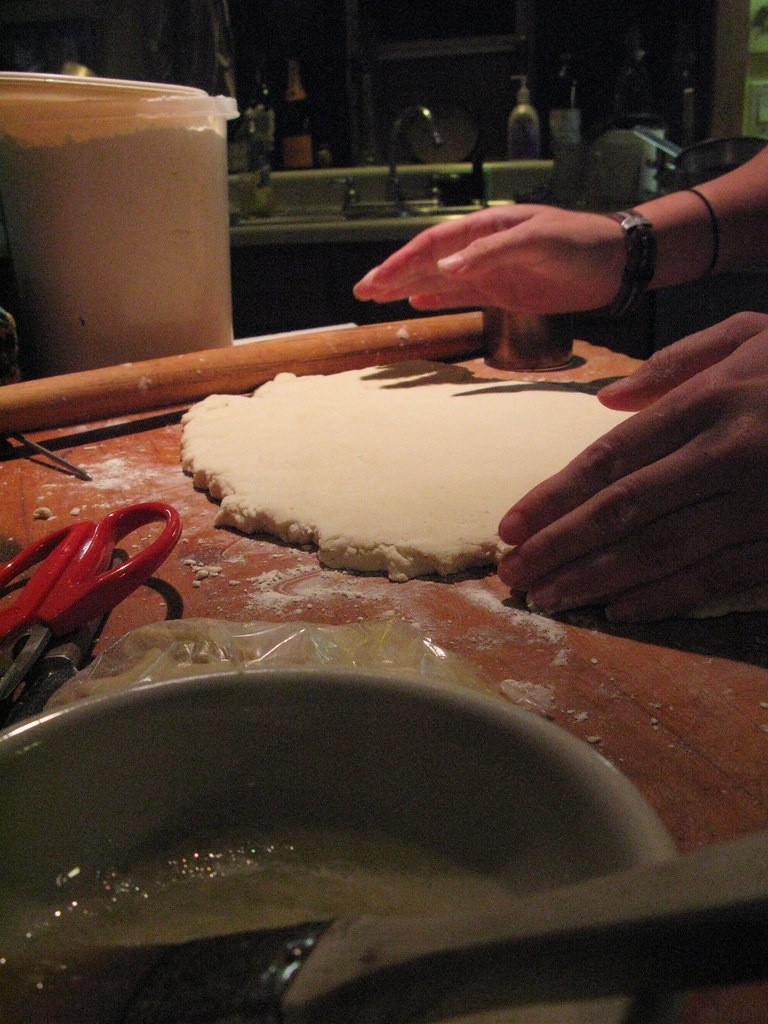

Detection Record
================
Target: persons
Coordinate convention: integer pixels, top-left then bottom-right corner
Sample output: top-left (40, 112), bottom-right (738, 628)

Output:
top-left (353, 147), bottom-right (768, 623)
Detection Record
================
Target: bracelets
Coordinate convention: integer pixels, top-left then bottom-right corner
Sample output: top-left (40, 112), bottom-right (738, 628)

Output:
top-left (686, 188), bottom-right (718, 279)
top-left (606, 208), bottom-right (656, 317)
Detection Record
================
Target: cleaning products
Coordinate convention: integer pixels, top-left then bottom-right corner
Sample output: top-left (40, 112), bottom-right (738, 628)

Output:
top-left (507, 74), bottom-right (541, 160)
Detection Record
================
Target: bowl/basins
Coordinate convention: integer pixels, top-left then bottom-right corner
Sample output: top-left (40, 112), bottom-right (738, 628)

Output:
top-left (0, 668), bottom-right (678, 1024)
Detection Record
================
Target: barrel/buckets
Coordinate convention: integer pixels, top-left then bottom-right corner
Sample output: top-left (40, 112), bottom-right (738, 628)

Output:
top-left (0, 72), bottom-right (238, 379)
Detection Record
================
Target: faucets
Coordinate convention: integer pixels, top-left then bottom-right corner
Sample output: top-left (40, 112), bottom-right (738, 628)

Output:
top-left (384, 104), bottom-right (444, 202)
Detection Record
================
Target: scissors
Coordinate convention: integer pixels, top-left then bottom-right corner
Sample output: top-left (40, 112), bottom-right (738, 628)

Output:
top-left (0, 500), bottom-right (183, 706)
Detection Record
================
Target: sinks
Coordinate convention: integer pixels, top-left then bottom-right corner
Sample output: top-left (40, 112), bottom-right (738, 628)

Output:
top-left (338, 201), bottom-right (422, 224)
top-left (398, 204), bottom-right (482, 219)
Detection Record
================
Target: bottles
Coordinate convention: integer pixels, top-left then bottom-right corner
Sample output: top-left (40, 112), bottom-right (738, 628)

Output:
top-left (281, 57), bottom-right (314, 170)
